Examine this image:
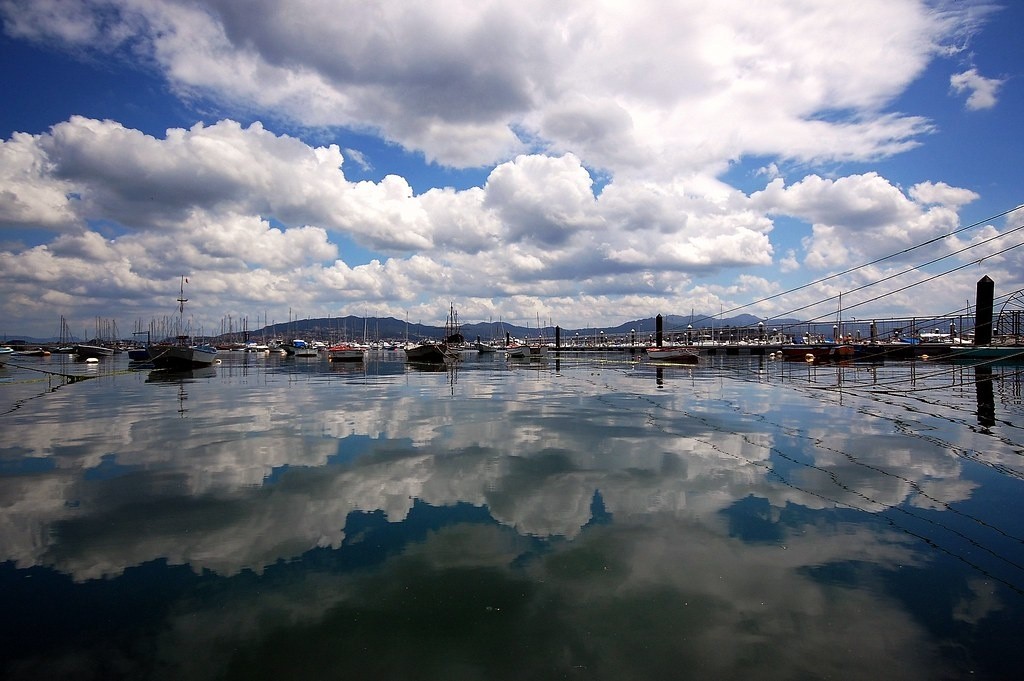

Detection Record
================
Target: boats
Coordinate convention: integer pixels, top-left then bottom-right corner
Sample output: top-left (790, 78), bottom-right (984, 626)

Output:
top-left (0, 274), bottom-right (979, 372)
